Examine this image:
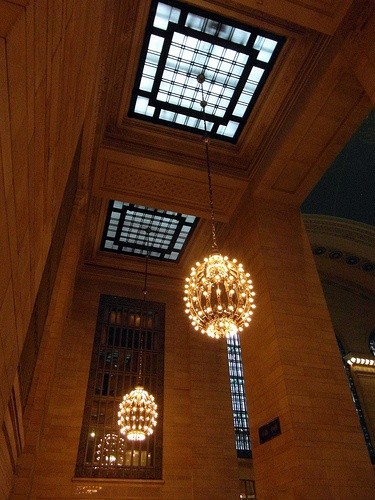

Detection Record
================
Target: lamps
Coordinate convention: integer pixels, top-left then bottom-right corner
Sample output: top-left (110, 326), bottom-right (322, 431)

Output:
top-left (117, 228), bottom-right (159, 442)
top-left (183, 73), bottom-right (255, 340)
